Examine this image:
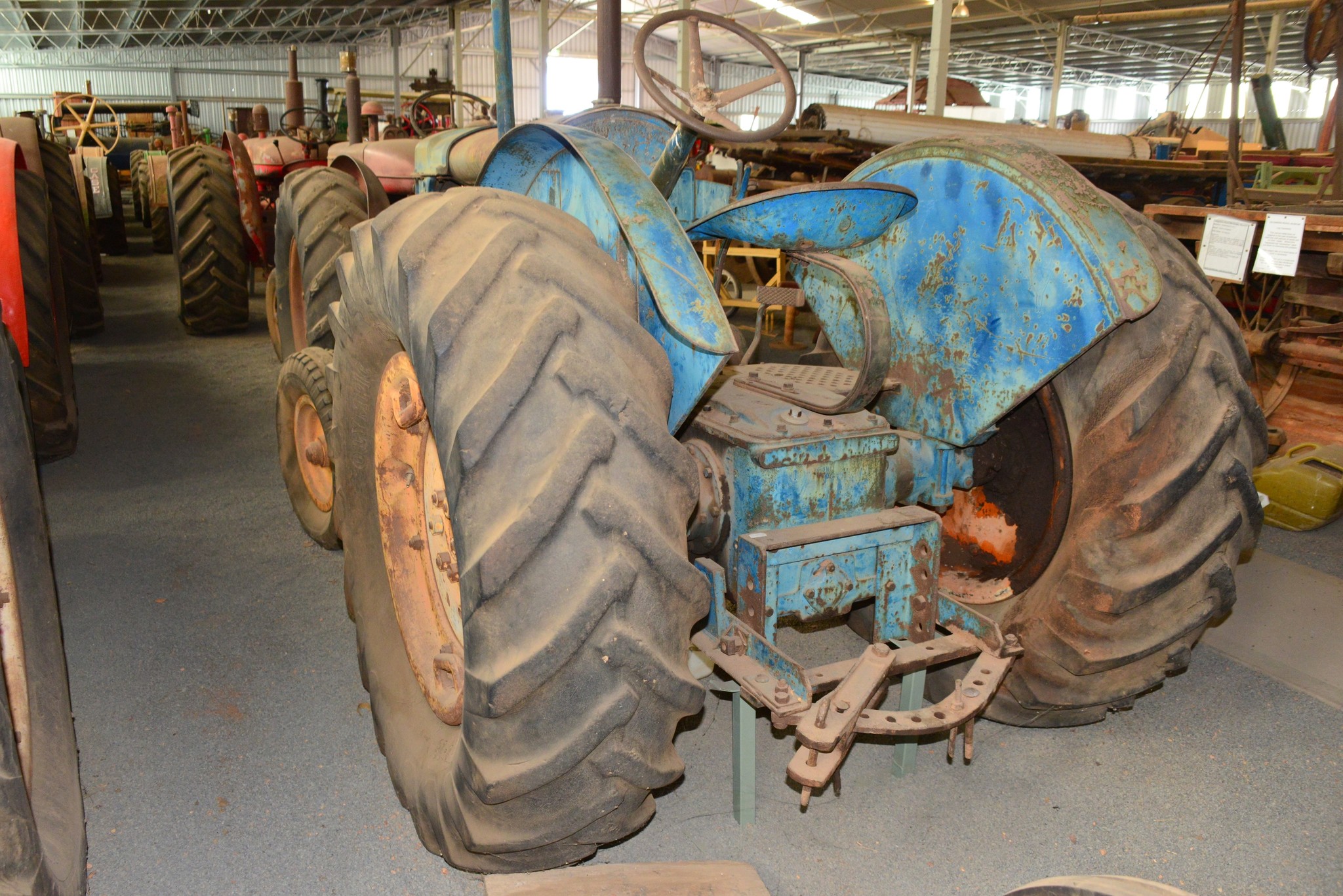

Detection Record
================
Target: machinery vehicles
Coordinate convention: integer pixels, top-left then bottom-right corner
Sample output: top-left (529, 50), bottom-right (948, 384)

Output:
top-left (0, 79), bottom-right (132, 896)
top-left (128, 8), bottom-right (1271, 876)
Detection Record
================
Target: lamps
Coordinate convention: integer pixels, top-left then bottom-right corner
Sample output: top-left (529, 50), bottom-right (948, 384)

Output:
top-left (952, 0), bottom-right (970, 17)
